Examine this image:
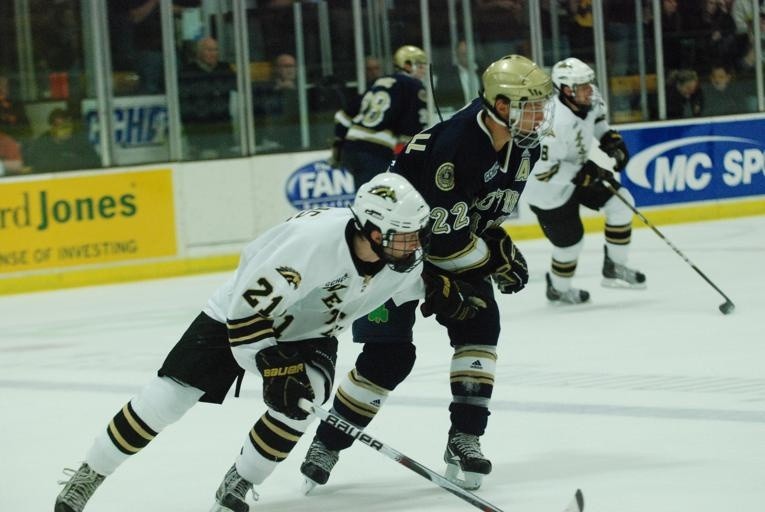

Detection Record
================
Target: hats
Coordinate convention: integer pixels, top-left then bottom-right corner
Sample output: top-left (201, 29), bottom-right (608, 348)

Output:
top-left (481, 52), bottom-right (555, 108)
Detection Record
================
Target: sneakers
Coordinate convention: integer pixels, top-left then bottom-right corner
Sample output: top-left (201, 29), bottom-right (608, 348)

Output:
top-left (602, 257), bottom-right (645, 285)
top-left (53, 460), bottom-right (106, 511)
top-left (443, 425), bottom-right (492, 474)
top-left (546, 286), bottom-right (590, 304)
top-left (215, 462), bottom-right (254, 511)
top-left (300, 433), bottom-right (340, 485)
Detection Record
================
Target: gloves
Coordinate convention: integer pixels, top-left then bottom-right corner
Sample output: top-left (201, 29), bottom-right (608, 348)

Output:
top-left (571, 161), bottom-right (617, 194)
top-left (251, 343), bottom-right (314, 420)
top-left (484, 222), bottom-right (529, 296)
top-left (599, 128), bottom-right (629, 173)
top-left (420, 272), bottom-right (488, 331)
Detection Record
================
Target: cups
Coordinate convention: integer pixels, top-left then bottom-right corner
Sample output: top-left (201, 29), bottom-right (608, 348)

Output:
top-left (47, 72), bottom-right (69, 98)
top-left (182, 8), bottom-right (201, 41)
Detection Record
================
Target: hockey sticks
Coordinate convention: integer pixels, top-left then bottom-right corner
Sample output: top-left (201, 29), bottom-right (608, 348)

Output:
top-left (297, 398), bottom-right (584, 510)
top-left (602, 179), bottom-right (736, 314)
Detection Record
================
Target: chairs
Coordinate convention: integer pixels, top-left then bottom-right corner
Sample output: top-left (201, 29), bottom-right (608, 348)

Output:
top-left (2, 3), bottom-right (763, 172)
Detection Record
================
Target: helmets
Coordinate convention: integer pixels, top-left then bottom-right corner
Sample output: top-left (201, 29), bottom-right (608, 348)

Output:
top-left (346, 170), bottom-right (432, 246)
top-left (550, 57), bottom-right (596, 97)
top-left (392, 44), bottom-right (428, 69)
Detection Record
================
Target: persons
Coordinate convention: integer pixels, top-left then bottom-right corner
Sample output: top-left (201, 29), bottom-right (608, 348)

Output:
top-left (301, 54), bottom-right (558, 485)
top-left (523, 56), bottom-right (647, 304)
top-left (53, 170), bottom-right (432, 512)
top-left (1, 0), bottom-right (765, 193)
